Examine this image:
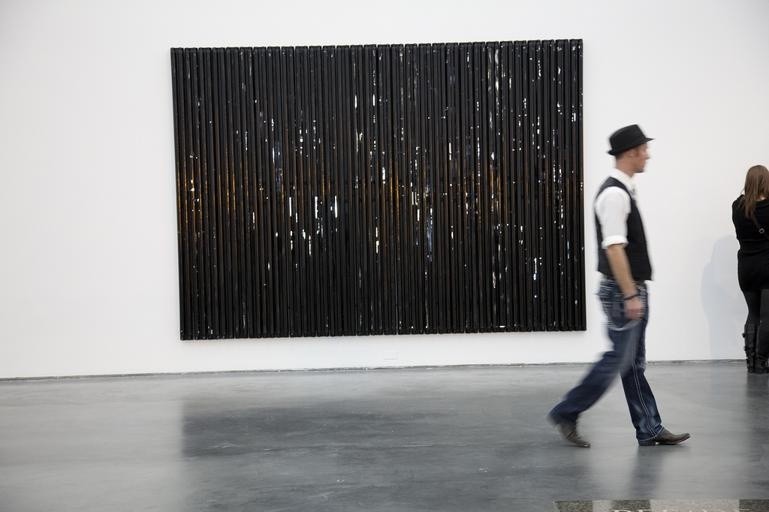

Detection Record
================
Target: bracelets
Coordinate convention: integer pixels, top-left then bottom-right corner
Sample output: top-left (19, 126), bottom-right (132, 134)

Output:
top-left (623, 294), bottom-right (640, 300)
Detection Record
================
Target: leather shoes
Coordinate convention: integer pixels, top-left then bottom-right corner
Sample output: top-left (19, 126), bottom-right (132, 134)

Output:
top-left (544, 410), bottom-right (593, 450)
top-left (634, 425), bottom-right (691, 448)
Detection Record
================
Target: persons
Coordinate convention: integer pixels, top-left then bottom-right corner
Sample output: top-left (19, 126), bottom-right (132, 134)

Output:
top-left (546, 125), bottom-right (691, 446)
top-left (731, 165), bottom-right (769, 374)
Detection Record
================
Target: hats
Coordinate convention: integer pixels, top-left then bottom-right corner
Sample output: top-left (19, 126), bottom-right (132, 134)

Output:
top-left (606, 123), bottom-right (654, 157)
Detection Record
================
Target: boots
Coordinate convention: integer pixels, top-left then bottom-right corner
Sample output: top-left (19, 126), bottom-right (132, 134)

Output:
top-left (742, 322), bottom-right (769, 374)
